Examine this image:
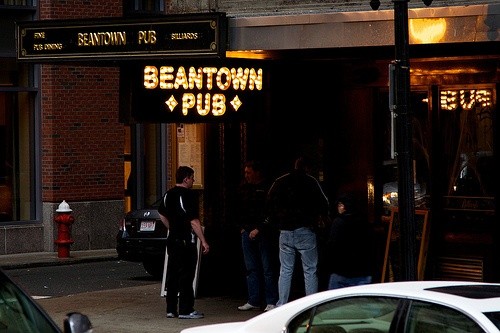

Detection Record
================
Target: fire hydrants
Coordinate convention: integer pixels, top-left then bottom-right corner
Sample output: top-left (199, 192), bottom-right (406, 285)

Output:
top-left (54, 199), bottom-right (76, 258)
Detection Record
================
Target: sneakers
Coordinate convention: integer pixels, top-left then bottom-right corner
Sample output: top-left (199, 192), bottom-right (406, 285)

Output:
top-left (264, 304), bottom-right (276, 312)
top-left (238, 302), bottom-right (261, 311)
top-left (166, 307), bottom-right (205, 319)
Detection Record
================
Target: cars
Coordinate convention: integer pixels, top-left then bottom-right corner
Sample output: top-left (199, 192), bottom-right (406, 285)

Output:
top-left (179, 280), bottom-right (500, 333)
top-left (366, 159), bottom-right (427, 210)
top-left (0, 270), bottom-right (95, 333)
top-left (114, 192), bottom-right (169, 279)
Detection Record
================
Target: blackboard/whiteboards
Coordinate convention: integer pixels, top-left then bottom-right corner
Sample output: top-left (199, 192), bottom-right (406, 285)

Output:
top-left (379, 207), bottom-right (429, 286)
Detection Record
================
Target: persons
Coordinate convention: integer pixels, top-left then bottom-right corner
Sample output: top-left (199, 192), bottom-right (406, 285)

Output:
top-left (265, 157), bottom-right (332, 306)
top-left (235, 160), bottom-right (278, 313)
top-left (156, 166), bottom-right (210, 320)
